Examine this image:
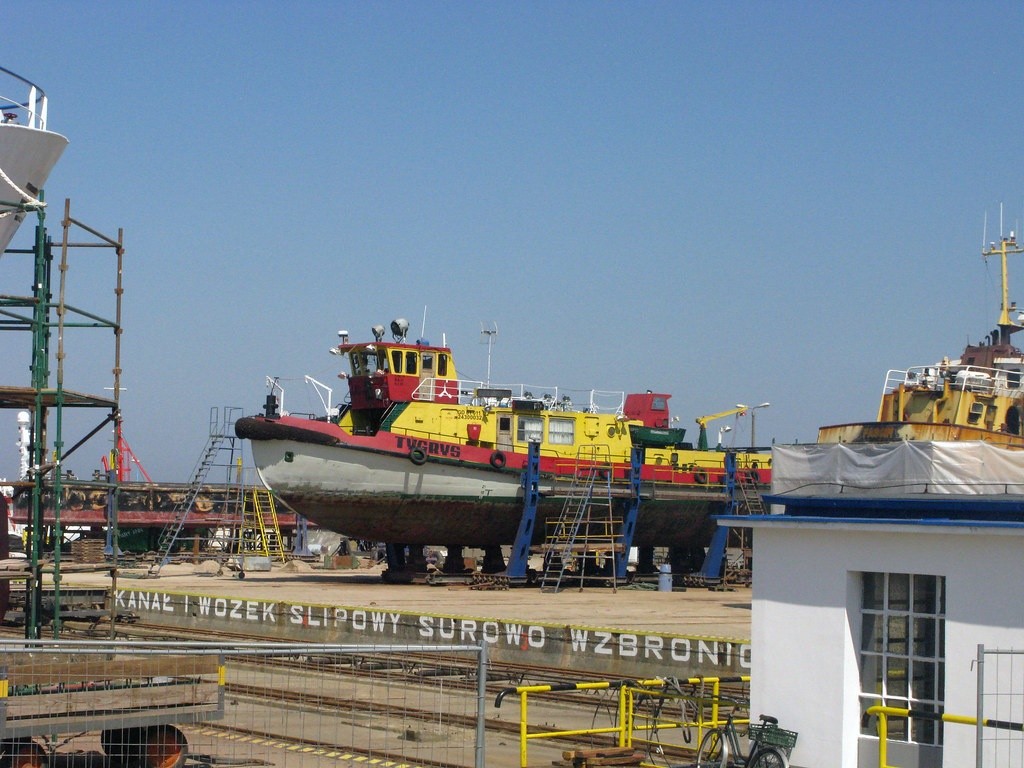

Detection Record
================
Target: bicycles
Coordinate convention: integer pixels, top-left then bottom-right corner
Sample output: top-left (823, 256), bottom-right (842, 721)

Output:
top-left (696, 695), bottom-right (798, 768)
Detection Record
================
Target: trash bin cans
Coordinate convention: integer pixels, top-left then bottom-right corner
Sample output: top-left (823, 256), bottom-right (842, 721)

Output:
top-left (658, 564), bottom-right (672, 592)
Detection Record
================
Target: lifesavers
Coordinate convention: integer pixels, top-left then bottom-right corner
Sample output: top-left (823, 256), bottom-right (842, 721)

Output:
top-left (490, 451), bottom-right (506, 468)
top-left (694, 468), bottom-right (707, 484)
top-left (746, 469), bottom-right (760, 484)
top-left (408, 447), bottom-right (427, 465)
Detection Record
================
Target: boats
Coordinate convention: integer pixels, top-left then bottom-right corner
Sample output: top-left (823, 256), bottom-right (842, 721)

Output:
top-left (770, 158), bottom-right (1024, 500)
top-left (0, 66), bottom-right (69, 257)
top-left (630, 424), bottom-right (686, 446)
top-left (234, 305), bottom-right (807, 551)
